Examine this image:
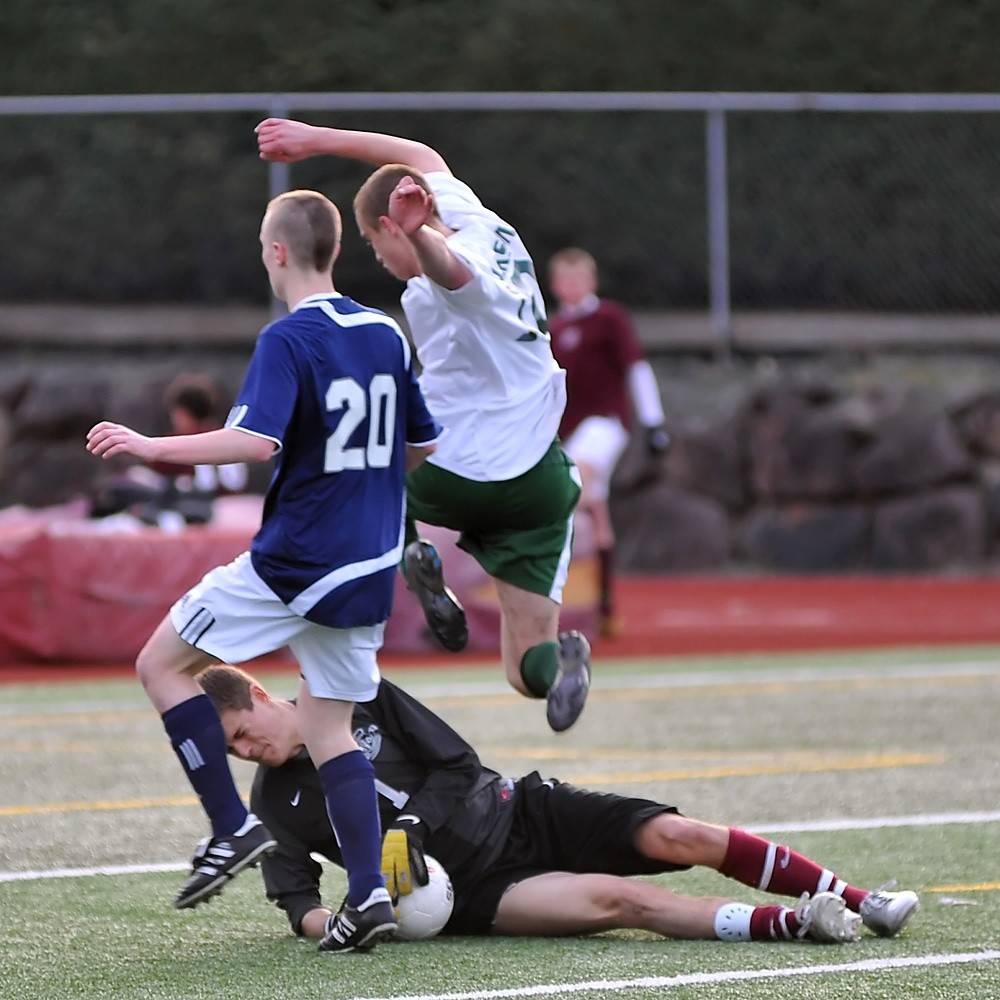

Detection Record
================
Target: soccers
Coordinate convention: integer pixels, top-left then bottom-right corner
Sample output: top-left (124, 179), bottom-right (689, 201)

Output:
top-left (388, 854), bottom-right (454, 940)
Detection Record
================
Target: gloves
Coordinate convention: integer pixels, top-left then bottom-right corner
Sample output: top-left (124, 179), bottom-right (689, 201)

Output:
top-left (646, 424), bottom-right (668, 454)
top-left (324, 891), bottom-right (351, 937)
top-left (379, 814), bottom-right (430, 906)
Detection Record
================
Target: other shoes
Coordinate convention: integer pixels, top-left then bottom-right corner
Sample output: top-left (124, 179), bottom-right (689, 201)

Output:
top-left (599, 611), bottom-right (622, 641)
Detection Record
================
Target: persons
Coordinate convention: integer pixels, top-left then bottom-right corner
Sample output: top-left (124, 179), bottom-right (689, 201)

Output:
top-left (120, 374), bottom-right (252, 518)
top-left (87, 189), bottom-right (446, 959)
top-left (253, 117), bottom-right (590, 733)
top-left (197, 666), bottom-right (918, 945)
top-left (540, 249), bottom-right (667, 638)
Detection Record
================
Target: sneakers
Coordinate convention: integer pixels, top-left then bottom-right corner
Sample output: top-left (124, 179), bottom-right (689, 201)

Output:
top-left (805, 894), bottom-right (860, 940)
top-left (178, 814), bottom-right (279, 911)
top-left (404, 539), bottom-right (470, 654)
top-left (314, 884), bottom-right (394, 954)
top-left (547, 632), bottom-right (593, 736)
top-left (862, 889), bottom-right (921, 938)
